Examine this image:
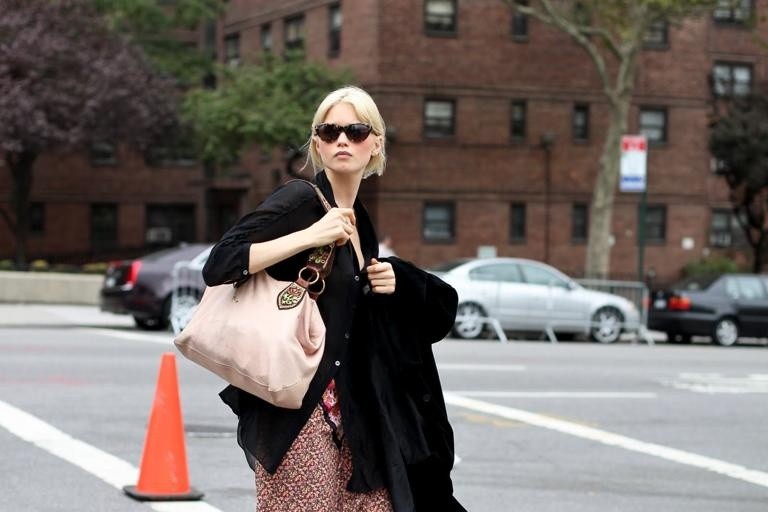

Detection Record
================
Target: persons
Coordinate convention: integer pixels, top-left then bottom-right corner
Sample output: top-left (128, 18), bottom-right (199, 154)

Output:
top-left (202, 86), bottom-right (407, 512)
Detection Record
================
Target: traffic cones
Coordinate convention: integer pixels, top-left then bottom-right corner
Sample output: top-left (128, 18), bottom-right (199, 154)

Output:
top-left (123, 355), bottom-right (206, 501)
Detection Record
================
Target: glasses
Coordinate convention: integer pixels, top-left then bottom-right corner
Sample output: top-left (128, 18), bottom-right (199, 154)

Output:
top-left (315, 123), bottom-right (373, 144)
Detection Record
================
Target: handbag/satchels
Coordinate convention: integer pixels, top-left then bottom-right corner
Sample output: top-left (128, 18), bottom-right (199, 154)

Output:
top-left (172, 262), bottom-right (327, 410)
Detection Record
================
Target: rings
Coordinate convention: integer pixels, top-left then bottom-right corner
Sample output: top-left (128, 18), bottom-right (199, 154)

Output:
top-left (344, 219), bottom-right (349, 225)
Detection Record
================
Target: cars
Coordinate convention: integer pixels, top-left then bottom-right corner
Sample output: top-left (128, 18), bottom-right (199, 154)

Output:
top-left (647, 271), bottom-right (768, 347)
top-left (100, 241), bottom-right (215, 329)
top-left (425, 256), bottom-right (640, 345)
